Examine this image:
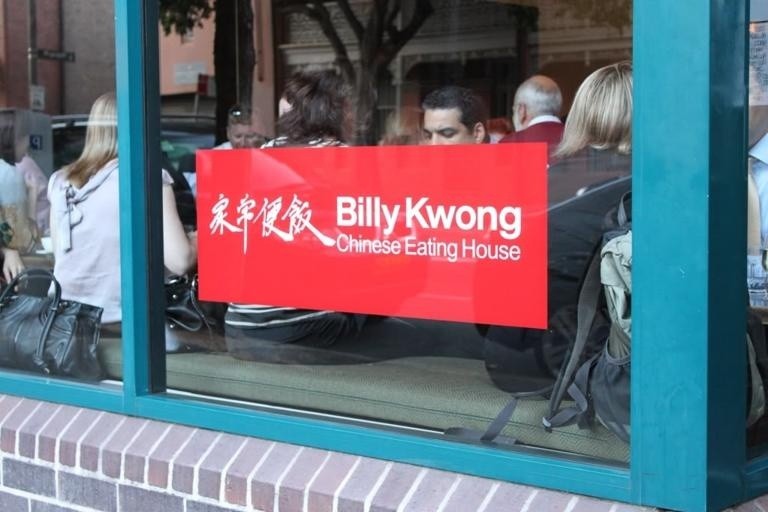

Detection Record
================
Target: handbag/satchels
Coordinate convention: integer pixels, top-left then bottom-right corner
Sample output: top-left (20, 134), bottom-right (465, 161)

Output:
top-left (0, 269), bottom-right (105, 384)
top-left (163, 273), bottom-right (226, 354)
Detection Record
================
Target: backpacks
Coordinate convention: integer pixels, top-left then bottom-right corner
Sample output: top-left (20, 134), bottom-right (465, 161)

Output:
top-left (542, 188), bottom-right (767, 446)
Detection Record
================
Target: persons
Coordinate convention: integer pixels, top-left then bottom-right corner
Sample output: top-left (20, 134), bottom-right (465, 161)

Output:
top-left (372, 74), bottom-right (566, 162)
top-left (0, 90), bottom-right (198, 358)
top-left (482, 57), bottom-right (636, 402)
top-left (223, 60), bottom-right (443, 367)
top-left (748, 29), bottom-right (768, 310)
top-left (210, 102), bottom-right (268, 150)
